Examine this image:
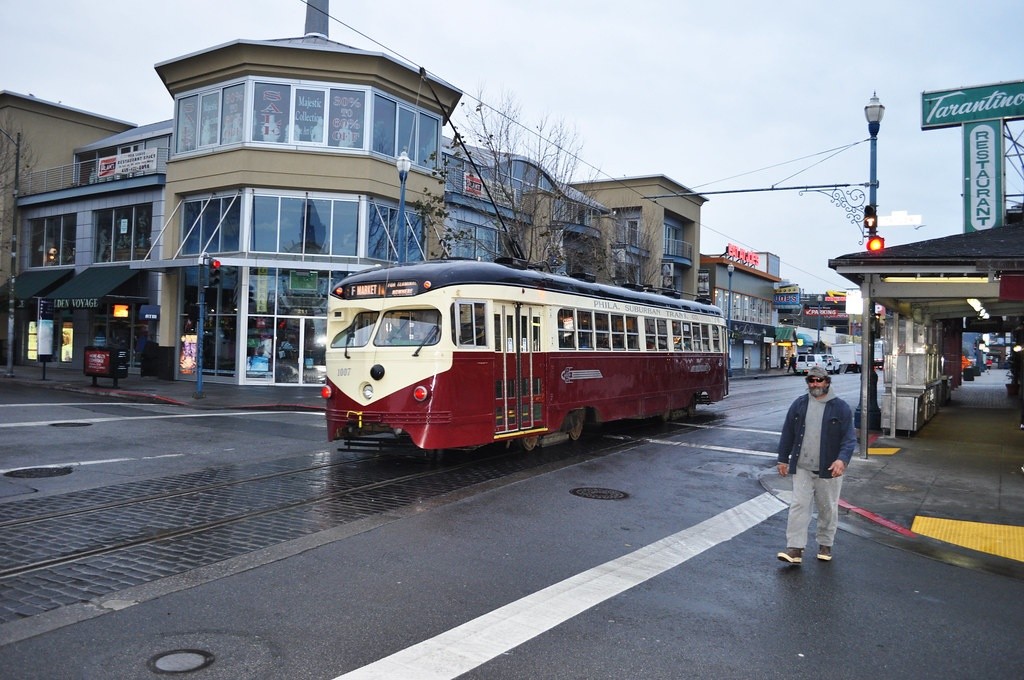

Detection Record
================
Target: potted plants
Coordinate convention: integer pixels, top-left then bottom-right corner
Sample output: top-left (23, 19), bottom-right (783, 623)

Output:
top-left (1005, 360), bottom-right (1020, 396)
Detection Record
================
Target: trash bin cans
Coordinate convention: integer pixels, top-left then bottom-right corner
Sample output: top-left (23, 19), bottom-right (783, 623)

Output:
top-left (84, 346), bottom-right (129, 379)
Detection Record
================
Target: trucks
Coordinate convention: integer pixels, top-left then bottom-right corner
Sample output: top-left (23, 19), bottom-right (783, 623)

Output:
top-left (831, 342), bottom-right (862, 374)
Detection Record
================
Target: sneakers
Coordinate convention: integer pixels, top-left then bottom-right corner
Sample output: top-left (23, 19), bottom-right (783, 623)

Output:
top-left (777, 547), bottom-right (802, 563)
top-left (817, 545), bottom-right (832, 560)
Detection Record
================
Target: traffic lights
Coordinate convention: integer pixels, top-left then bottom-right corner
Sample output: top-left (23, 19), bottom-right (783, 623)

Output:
top-left (866, 234), bottom-right (885, 251)
top-left (209, 258), bottom-right (220, 287)
top-left (862, 204), bottom-right (878, 228)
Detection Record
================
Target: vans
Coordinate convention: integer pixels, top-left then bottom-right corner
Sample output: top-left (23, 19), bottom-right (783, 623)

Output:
top-left (795, 353), bottom-right (841, 375)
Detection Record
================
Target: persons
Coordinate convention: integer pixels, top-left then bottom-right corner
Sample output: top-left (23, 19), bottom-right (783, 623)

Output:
top-left (600, 334), bottom-right (620, 348)
top-left (580, 338), bottom-right (589, 348)
top-left (401, 320), bottom-right (415, 340)
top-left (986, 357), bottom-right (992, 375)
top-left (787, 354), bottom-right (796, 374)
top-left (646, 342), bottom-right (655, 349)
top-left (777, 366), bottom-right (858, 565)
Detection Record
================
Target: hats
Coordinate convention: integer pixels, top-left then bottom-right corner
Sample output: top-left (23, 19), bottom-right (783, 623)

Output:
top-left (808, 366), bottom-right (828, 378)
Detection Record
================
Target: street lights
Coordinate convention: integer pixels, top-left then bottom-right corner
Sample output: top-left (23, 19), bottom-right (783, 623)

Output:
top-left (817, 294), bottom-right (823, 353)
top-left (725, 262), bottom-right (735, 377)
top-left (853, 87), bottom-right (885, 432)
top-left (396, 150), bottom-right (411, 263)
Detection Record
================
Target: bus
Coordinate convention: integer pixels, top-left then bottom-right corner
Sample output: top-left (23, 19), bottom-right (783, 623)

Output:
top-left (321, 65), bottom-right (731, 455)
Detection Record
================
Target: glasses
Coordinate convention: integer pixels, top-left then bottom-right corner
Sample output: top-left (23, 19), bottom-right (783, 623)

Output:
top-left (808, 377), bottom-right (824, 383)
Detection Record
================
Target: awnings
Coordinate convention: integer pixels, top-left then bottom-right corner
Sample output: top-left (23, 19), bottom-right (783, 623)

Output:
top-left (0, 269), bottom-right (75, 309)
top-left (139, 304), bottom-right (160, 321)
top-left (44, 265), bottom-right (140, 308)
top-left (797, 334), bottom-right (813, 346)
